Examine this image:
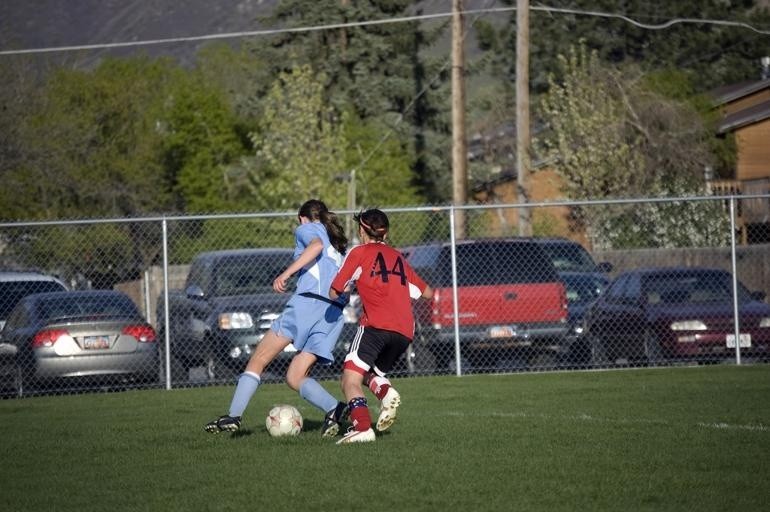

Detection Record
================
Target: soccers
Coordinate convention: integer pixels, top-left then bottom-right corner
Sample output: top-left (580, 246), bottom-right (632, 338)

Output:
top-left (265, 404), bottom-right (303, 436)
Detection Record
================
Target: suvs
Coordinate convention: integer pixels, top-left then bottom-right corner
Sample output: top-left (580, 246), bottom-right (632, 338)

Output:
top-left (402, 240), bottom-right (578, 373)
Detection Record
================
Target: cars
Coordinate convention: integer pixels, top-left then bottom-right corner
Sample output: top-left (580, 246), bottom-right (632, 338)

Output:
top-left (538, 242), bottom-right (616, 325)
top-left (4, 290), bottom-right (159, 390)
top-left (583, 266), bottom-right (767, 367)
top-left (2, 272), bottom-right (72, 333)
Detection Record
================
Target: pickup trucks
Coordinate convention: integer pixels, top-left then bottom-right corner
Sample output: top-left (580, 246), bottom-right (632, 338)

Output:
top-left (154, 248), bottom-right (301, 374)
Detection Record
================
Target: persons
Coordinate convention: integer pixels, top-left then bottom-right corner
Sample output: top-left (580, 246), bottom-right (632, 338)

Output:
top-left (326, 205), bottom-right (434, 445)
top-left (202, 198), bottom-right (350, 441)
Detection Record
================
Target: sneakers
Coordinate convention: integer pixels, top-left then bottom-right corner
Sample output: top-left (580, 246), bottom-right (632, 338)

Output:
top-left (204, 414), bottom-right (241, 436)
top-left (322, 400), bottom-right (349, 439)
top-left (336, 427), bottom-right (378, 444)
top-left (375, 387), bottom-right (401, 432)
